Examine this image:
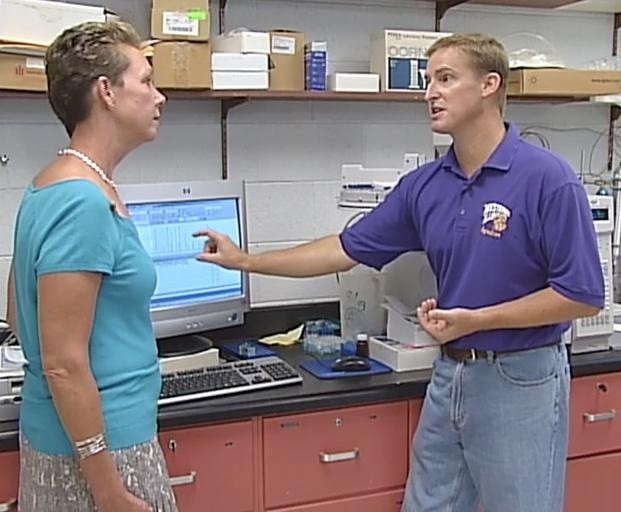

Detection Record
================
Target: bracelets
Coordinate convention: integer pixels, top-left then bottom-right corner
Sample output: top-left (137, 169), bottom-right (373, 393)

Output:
top-left (68, 430), bottom-right (110, 461)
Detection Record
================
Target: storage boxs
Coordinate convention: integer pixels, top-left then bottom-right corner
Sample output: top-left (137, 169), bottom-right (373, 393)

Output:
top-left (0, 0), bottom-right (104, 94)
top-left (368, 25), bottom-right (454, 95)
top-left (141, 0), bottom-right (305, 95)
top-left (506, 66), bottom-right (621, 100)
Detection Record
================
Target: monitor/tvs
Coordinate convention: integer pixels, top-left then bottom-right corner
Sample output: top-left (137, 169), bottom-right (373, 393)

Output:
top-left (114, 179), bottom-right (251, 359)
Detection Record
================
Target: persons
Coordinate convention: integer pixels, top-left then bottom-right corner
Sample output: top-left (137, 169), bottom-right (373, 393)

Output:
top-left (189, 29), bottom-right (608, 511)
top-left (0, 17), bottom-right (183, 512)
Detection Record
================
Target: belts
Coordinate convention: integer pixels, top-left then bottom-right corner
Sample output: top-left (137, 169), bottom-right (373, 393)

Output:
top-left (440, 339), bottom-right (562, 363)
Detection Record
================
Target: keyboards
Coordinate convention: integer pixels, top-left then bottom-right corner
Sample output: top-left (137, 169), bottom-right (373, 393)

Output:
top-left (157, 355), bottom-right (303, 406)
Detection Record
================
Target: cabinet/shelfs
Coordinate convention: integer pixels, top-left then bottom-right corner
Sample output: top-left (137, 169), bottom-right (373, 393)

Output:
top-left (561, 372), bottom-right (621, 512)
top-left (157, 402), bottom-right (411, 511)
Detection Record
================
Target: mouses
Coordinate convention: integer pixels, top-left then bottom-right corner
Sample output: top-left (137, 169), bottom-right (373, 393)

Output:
top-left (331, 355), bottom-right (371, 373)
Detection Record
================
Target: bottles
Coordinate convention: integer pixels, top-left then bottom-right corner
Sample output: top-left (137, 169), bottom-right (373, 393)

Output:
top-left (355, 332), bottom-right (371, 361)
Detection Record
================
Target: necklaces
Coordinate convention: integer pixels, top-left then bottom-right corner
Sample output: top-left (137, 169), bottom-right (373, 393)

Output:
top-left (55, 144), bottom-right (118, 192)
